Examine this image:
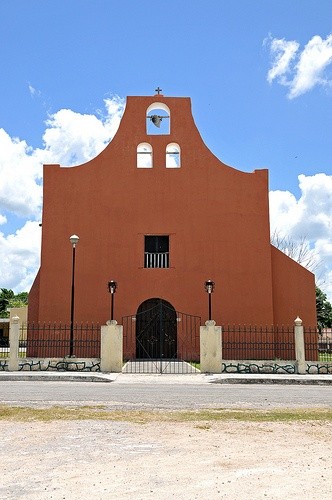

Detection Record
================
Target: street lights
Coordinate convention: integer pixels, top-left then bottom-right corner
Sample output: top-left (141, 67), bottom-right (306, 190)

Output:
top-left (69, 234), bottom-right (79, 356)
top-left (204, 279), bottom-right (215, 320)
top-left (107, 280), bottom-right (117, 320)
